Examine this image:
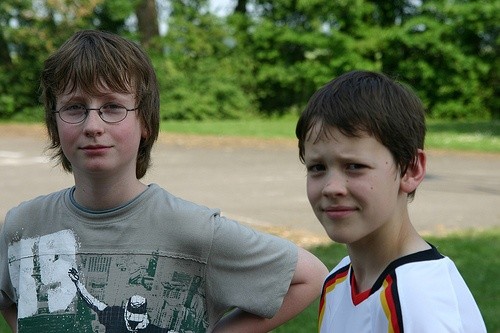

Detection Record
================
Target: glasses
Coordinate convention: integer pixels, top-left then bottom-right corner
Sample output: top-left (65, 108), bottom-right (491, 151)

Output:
top-left (52, 100), bottom-right (141, 124)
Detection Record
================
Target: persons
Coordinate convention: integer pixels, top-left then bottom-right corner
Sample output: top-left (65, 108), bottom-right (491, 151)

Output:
top-left (295, 68), bottom-right (488, 333)
top-left (0, 31), bottom-right (331, 333)
